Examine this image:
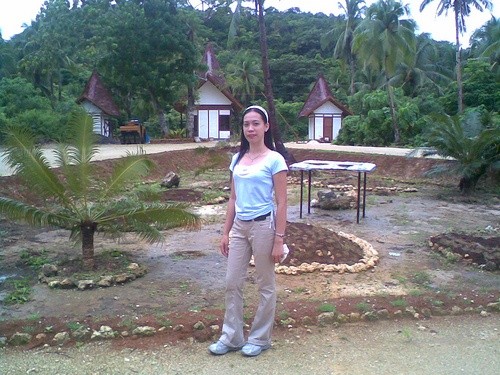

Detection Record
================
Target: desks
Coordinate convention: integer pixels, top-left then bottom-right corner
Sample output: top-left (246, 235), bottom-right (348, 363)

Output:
top-left (289, 160), bottom-right (376, 224)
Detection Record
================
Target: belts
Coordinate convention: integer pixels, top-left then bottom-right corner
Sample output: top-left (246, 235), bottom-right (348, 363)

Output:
top-left (239, 211), bottom-right (272, 223)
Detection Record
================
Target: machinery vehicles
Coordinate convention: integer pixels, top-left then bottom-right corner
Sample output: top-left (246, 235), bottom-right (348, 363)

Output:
top-left (118, 119), bottom-right (147, 144)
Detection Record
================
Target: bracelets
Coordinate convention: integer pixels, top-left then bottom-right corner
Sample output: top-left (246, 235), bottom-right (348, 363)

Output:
top-left (275, 232), bottom-right (286, 238)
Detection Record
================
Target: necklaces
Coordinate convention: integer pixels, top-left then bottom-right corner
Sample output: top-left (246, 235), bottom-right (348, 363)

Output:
top-left (242, 145), bottom-right (267, 162)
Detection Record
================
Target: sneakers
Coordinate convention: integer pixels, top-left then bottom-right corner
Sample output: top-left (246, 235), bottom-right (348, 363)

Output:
top-left (242, 340), bottom-right (271, 356)
top-left (209, 339), bottom-right (245, 354)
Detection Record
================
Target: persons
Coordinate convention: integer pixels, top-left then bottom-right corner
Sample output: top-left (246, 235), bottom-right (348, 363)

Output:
top-left (209, 105), bottom-right (289, 356)
